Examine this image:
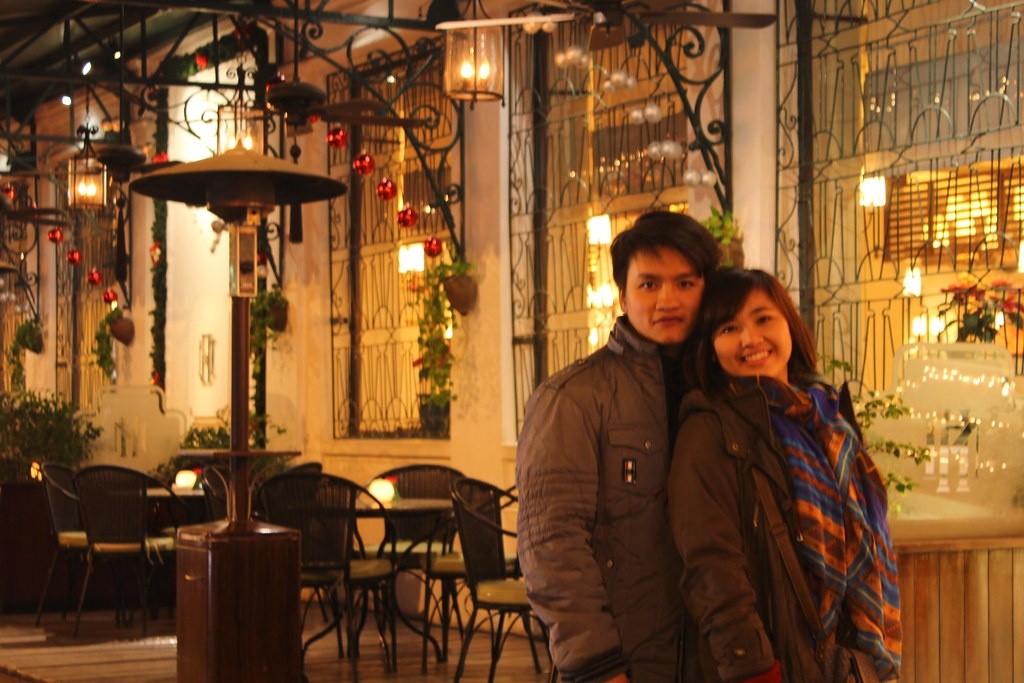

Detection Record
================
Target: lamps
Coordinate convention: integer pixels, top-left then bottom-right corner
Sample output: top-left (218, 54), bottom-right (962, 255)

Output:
top-left (129, 136), bottom-right (348, 299)
top-left (441, 0), bottom-right (506, 110)
top-left (217, 13), bottom-right (266, 157)
top-left (67, 80), bottom-right (107, 208)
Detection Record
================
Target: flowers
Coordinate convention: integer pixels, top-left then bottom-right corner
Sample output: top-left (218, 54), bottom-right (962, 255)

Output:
top-left (936, 280), bottom-right (1024, 344)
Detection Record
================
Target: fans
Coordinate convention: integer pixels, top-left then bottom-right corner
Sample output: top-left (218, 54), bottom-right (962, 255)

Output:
top-left (434, 0), bottom-right (777, 49)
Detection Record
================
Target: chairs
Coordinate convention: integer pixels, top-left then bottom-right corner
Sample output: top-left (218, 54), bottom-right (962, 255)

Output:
top-left (32, 462), bottom-right (553, 683)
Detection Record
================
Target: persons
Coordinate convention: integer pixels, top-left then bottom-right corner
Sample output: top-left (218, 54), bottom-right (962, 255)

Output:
top-left (667, 269), bottom-right (904, 683)
top-left (515, 212), bottom-right (722, 683)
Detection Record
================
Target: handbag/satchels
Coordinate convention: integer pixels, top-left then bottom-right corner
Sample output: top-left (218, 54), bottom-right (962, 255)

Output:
top-left (814, 640), bottom-right (881, 683)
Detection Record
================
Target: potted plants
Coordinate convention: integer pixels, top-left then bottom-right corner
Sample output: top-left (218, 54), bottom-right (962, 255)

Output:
top-left (702, 206), bottom-right (745, 270)
top-left (6, 318), bottom-right (43, 397)
top-left (415, 256), bottom-right (477, 413)
top-left (90, 307), bottom-right (133, 381)
top-left (248, 285), bottom-right (289, 444)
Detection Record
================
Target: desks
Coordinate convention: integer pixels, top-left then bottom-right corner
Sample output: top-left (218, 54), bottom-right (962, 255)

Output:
top-left (340, 498), bottom-right (454, 677)
top-left (139, 484), bottom-right (208, 621)
